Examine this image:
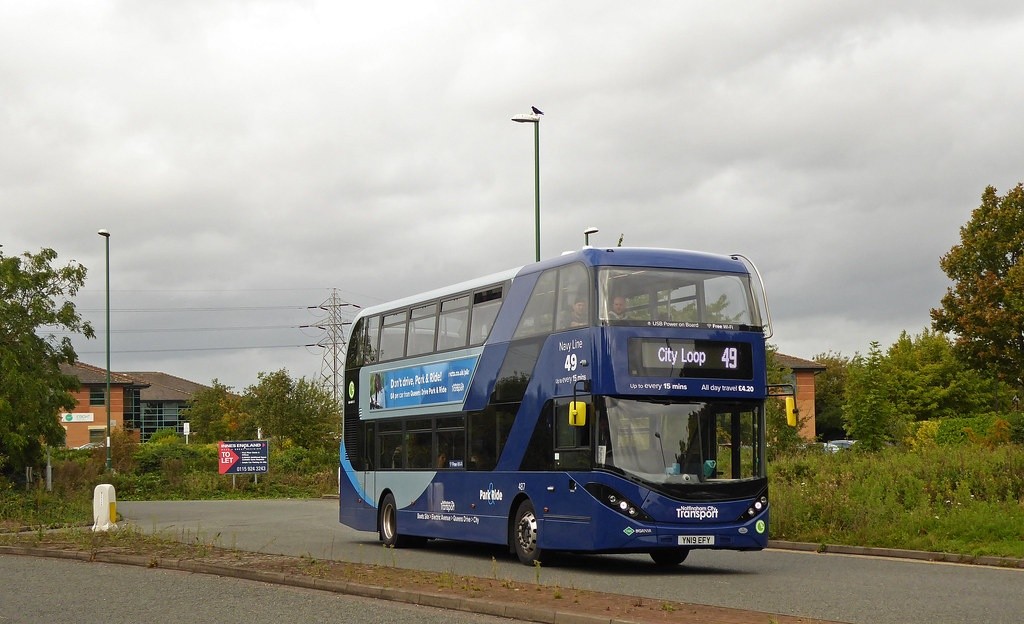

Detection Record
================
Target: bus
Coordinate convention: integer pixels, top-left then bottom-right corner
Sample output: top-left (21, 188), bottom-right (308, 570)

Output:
top-left (335, 247), bottom-right (797, 568)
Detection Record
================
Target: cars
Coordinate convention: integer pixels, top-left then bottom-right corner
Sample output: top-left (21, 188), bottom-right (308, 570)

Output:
top-left (796, 439), bottom-right (861, 454)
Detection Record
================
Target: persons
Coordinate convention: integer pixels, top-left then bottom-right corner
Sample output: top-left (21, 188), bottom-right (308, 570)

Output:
top-left (565, 298), bottom-right (589, 329)
top-left (609, 295), bottom-right (630, 321)
top-left (437, 452), bottom-right (447, 469)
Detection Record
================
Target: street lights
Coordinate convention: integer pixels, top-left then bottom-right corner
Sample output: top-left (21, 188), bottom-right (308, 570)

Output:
top-left (510, 113), bottom-right (541, 263)
top-left (97, 228), bottom-right (111, 472)
top-left (583, 227), bottom-right (599, 246)
